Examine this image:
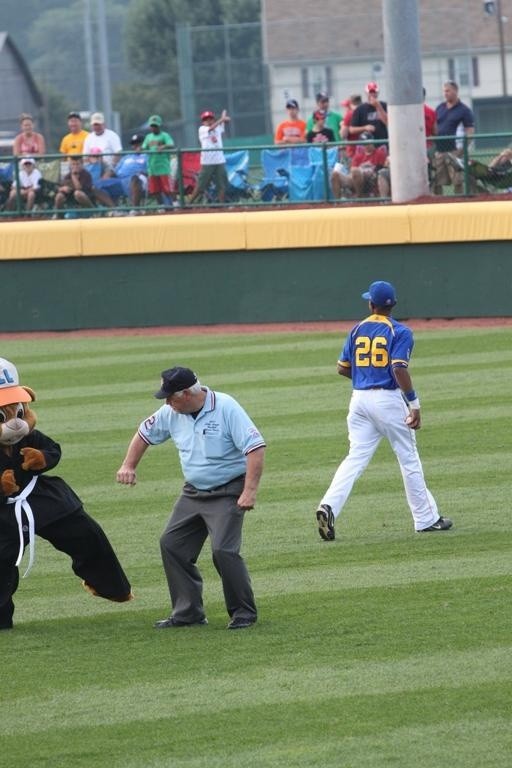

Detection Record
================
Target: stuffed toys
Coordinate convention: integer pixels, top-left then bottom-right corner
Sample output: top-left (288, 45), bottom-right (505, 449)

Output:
top-left (0, 358), bottom-right (133, 629)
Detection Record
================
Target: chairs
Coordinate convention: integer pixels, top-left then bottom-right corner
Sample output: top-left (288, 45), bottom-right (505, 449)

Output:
top-left (208, 150), bottom-right (249, 201)
top-left (260, 149), bottom-right (289, 202)
top-left (308, 147), bottom-right (338, 201)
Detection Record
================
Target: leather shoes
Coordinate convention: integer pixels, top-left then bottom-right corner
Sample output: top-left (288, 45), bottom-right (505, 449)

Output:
top-left (154, 617), bottom-right (209, 629)
top-left (228, 616), bottom-right (257, 628)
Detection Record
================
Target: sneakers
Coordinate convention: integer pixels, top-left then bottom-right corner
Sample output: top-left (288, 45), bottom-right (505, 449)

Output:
top-left (316, 504), bottom-right (335, 540)
top-left (416, 517), bottom-right (453, 533)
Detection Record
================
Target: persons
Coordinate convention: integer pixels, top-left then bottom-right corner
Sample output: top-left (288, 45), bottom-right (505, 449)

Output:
top-left (137, 115), bottom-right (180, 213)
top-left (423, 88), bottom-right (439, 191)
top-left (116, 366), bottom-right (267, 629)
top-left (60, 112), bottom-right (91, 155)
top-left (331, 132), bottom-right (386, 199)
top-left (274, 99), bottom-right (307, 145)
top-left (84, 147), bottom-right (116, 208)
top-left (340, 94), bottom-right (362, 157)
top-left (13, 114), bottom-right (45, 155)
top-left (348, 83), bottom-right (390, 157)
top-left (305, 93), bottom-right (343, 142)
top-left (305, 110), bottom-right (336, 143)
top-left (468, 141), bottom-right (512, 196)
top-left (433, 80), bottom-right (475, 196)
top-left (187, 109), bottom-right (230, 204)
top-left (316, 281), bottom-right (453, 540)
top-left (51, 156), bottom-right (99, 218)
top-left (90, 134), bottom-right (150, 217)
top-left (82, 113), bottom-right (122, 168)
top-left (377, 156), bottom-right (390, 198)
top-left (6, 159), bottom-right (46, 211)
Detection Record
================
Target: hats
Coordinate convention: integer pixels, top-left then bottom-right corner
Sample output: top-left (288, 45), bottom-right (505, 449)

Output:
top-left (90, 112), bottom-right (105, 125)
top-left (154, 367), bottom-right (197, 399)
top-left (350, 94), bottom-right (362, 105)
top-left (67, 111), bottom-right (80, 119)
top-left (361, 281), bottom-right (395, 306)
top-left (0, 357), bottom-right (32, 407)
top-left (365, 82), bottom-right (379, 93)
top-left (315, 92), bottom-right (329, 101)
top-left (21, 157), bottom-right (35, 164)
top-left (200, 111), bottom-right (214, 119)
top-left (128, 133), bottom-right (144, 144)
top-left (359, 131), bottom-right (374, 140)
top-left (148, 115), bottom-right (161, 126)
top-left (286, 99), bottom-right (297, 108)
top-left (314, 110), bottom-right (326, 120)
